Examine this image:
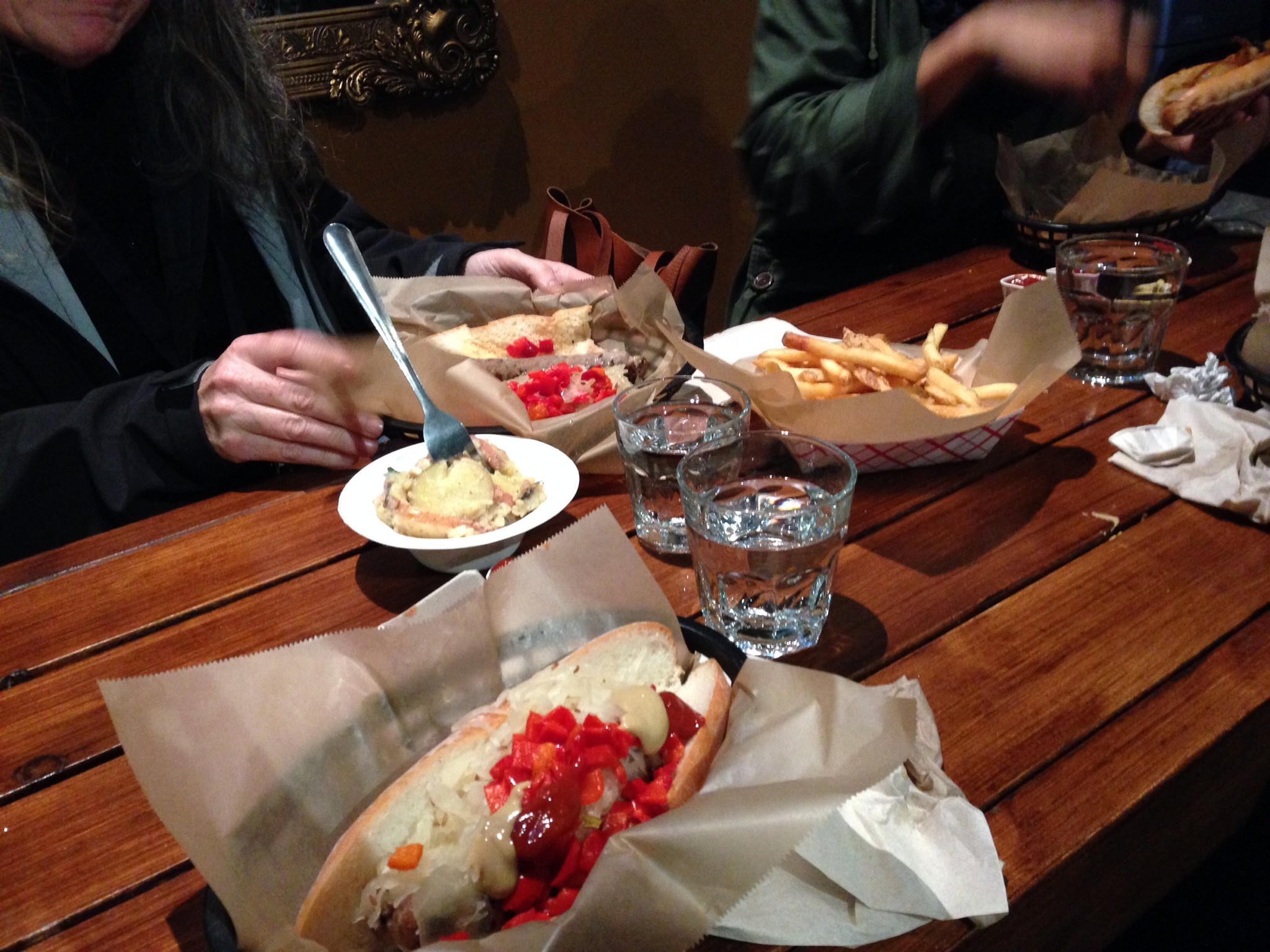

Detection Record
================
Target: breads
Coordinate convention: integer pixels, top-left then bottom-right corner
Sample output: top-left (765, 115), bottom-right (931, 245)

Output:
top-left (1139, 55), bottom-right (1270, 137)
top-left (293, 621), bottom-right (732, 952)
top-left (422, 304), bottom-right (604, 360)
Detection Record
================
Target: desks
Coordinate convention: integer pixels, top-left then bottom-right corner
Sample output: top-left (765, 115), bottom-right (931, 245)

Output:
top-left (0, 234), bottom-right (1270, 952)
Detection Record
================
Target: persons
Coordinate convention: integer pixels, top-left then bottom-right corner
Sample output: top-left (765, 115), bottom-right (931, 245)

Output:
top-left (0, 0), bottom-right (598, 562)
top-left (728, 0), bottom-right (1270, 330)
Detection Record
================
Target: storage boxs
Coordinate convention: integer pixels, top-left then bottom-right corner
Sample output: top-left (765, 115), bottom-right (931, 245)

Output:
top-left (765, 407), bottom-right (1025, 474)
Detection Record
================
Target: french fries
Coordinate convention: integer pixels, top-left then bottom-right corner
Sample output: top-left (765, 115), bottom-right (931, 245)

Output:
top-left (753, 323), bottom-right (1019, 418)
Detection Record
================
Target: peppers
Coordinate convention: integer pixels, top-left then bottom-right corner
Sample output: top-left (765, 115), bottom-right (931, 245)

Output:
top-left (506, 337), bottom-right (554, 358)
top-left (508, 362), bottom-right (618, 421)
top-left (388, 707), bottom-right (684, 944)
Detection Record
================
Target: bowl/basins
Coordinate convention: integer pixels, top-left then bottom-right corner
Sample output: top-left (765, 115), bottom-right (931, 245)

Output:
top-left (337, 433), bottom-right (581, 573)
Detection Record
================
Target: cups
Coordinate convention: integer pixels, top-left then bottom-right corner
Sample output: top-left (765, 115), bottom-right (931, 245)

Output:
top-left (1045, 266), bottom-right (1101, 293)
top-left (1055, 232), bottom-right (1188, 384)
top-left (612, 374), bottom-right (751, 554)
top-left (676, 429), bottom-right (859, 660)
top-left (1000, 273), bottom-right (1048, 298)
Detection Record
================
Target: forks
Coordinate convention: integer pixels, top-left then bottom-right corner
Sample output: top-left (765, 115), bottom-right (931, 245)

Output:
top-left (323, 222), bottom-right (491, 473)
